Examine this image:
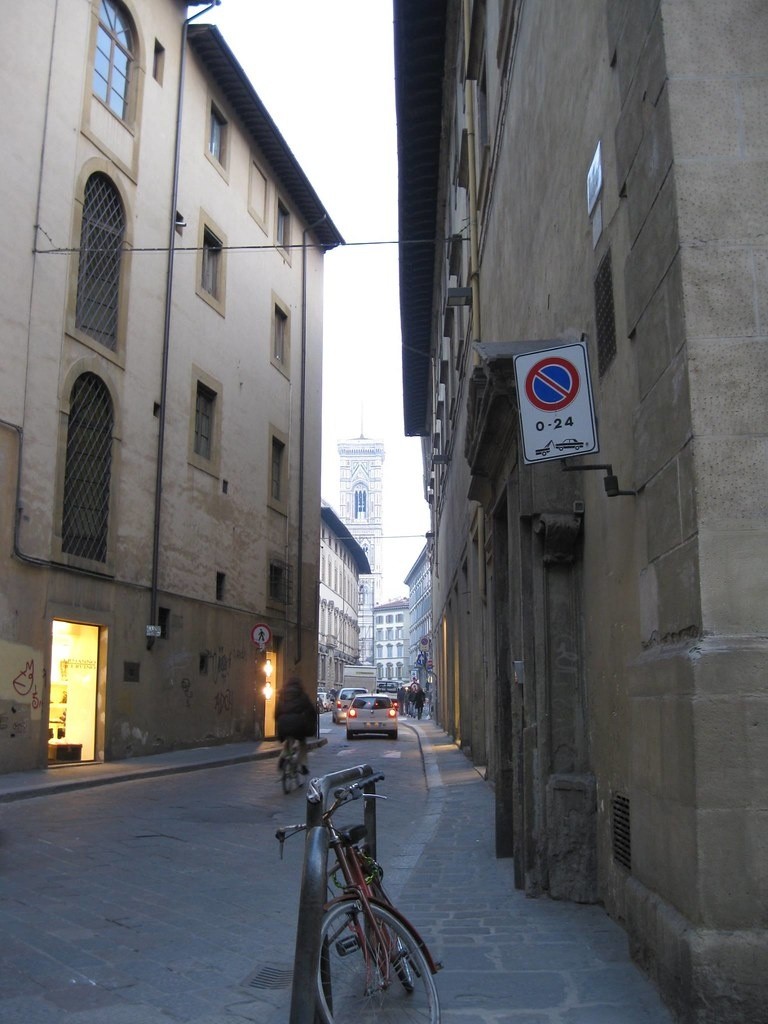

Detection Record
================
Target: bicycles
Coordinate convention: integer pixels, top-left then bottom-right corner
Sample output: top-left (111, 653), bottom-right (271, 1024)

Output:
top-left (278, 739), bottom-right (310, 794)
top-left (275, 768), bottom-right (446, 1024)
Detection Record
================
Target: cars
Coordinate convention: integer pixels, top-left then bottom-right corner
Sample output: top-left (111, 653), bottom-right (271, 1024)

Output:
top-left (330, 688), bottom-right (368, 725)
top-left (343, 694), bottom-right (399, 740)
top-left (317, 692), bottom-right (333, 711)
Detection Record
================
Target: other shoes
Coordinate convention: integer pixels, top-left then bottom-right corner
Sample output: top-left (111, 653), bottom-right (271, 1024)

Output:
top-left (277, 749), bottom-right (288, 770)
top-left (300, 755), bottom-right (310, 775)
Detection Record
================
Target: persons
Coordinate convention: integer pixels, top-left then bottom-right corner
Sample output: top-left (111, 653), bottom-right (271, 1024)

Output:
top-left (397, 686), bottom-right (425, 720)
top-left (276, 678), bottom-right (315, 774)
top-left (329, 688), bottom-right (340, 704)
